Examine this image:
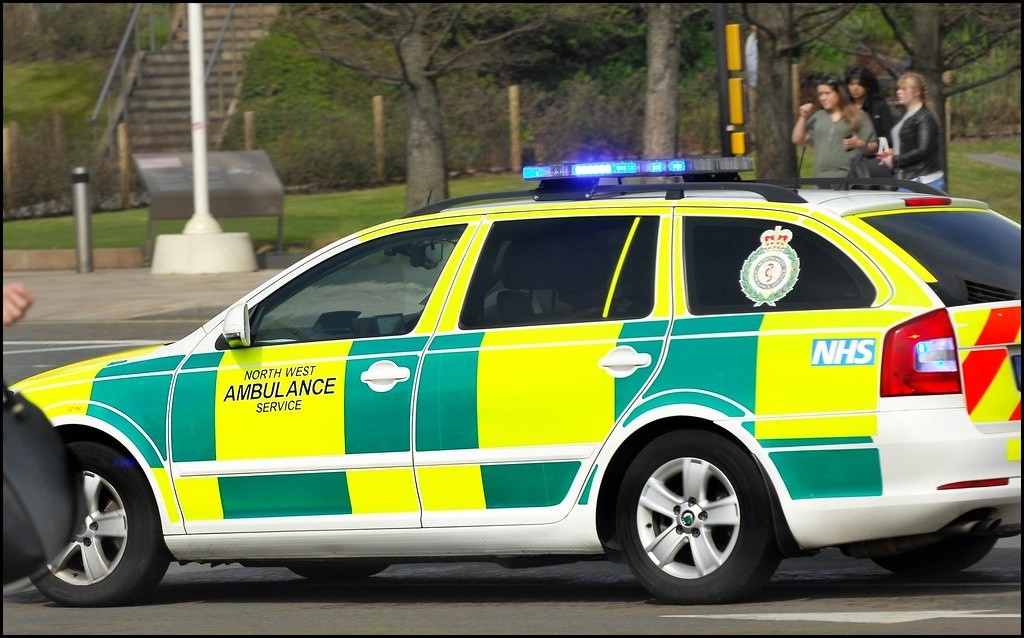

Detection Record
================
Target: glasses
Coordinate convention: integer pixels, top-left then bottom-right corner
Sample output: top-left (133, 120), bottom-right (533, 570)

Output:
top-left (814, 77), bottom-right (839, 92)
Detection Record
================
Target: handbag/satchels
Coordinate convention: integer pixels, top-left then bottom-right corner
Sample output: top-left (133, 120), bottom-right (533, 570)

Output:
top-left (850, 136), bottom-right (899, 191)
top-left (0, 381), bottom-right (80, 586)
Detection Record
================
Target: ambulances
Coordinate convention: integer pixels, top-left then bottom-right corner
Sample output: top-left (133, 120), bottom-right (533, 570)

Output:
top-left (9, 154), bottom-right (1024, 606)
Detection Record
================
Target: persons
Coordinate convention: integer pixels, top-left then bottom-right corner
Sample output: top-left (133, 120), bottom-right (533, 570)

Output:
top-left (843, 65), bottom-right (894, 192)
top-left (791, 77), bottom-right (879, 189)
top-left (875, 73), bottom-right (946, 193)
